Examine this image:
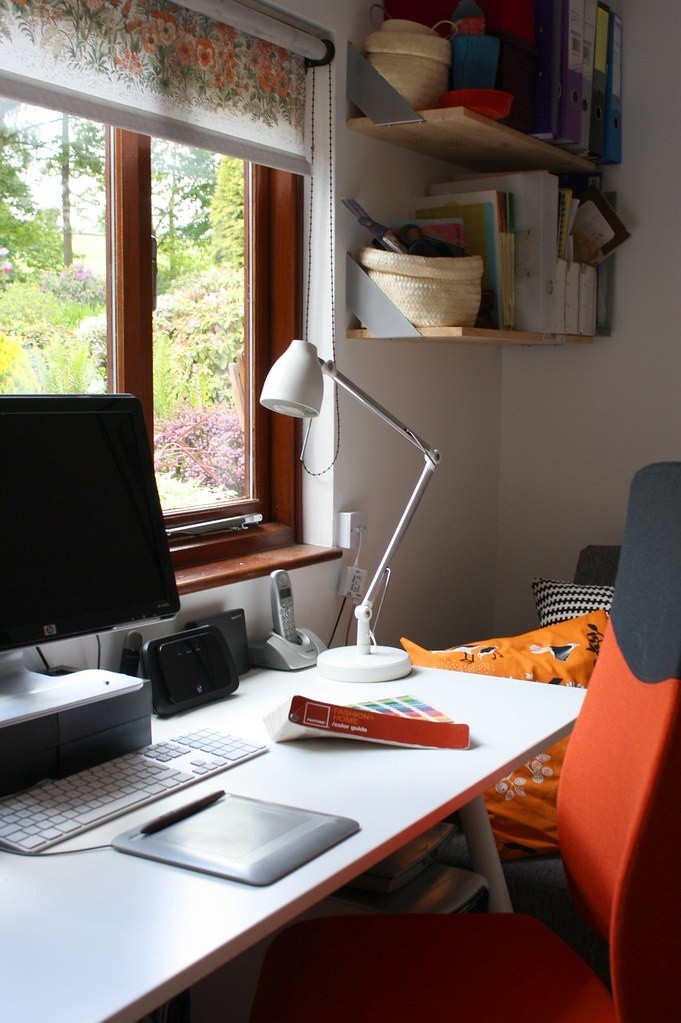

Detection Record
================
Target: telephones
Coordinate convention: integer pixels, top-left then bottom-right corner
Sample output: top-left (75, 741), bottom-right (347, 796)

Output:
top-left (261, 568), bottom-right (329, 671)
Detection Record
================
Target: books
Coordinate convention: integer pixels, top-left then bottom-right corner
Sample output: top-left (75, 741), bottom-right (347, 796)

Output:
top-left (410, 0), bottom-right (633, 337)
top-left (262, 693), bottom-right (470, 750)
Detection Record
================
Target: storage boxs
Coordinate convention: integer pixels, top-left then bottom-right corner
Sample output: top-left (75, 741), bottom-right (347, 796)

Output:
top-left (383, 0), bottom-right (624, 166)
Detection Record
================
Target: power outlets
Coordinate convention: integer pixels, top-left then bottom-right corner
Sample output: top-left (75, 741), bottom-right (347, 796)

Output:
top-left (338, 510), bottom-right (369, 550)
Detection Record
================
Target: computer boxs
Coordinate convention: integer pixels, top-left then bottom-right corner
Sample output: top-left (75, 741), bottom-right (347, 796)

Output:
top-left (186, 865), bottom-right (493, 1023)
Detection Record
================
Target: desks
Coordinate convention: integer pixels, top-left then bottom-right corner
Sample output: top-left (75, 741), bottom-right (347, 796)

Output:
top-left (0, 651), bottom-right (589, 1023)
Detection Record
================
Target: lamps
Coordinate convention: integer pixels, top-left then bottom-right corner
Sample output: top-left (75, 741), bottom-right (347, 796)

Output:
top-left (258, 339), bottom-right (443, 686)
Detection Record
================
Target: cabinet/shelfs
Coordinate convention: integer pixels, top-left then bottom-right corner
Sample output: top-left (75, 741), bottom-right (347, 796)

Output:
top-left (346, 41), bottom-right (596, 348)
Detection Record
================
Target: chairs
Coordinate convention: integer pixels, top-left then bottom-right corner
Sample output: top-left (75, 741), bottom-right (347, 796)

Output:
top-left (237, 459), bottom-right (681, 1023)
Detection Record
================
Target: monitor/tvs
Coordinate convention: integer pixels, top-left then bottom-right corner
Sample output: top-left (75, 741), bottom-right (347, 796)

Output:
top-left (0, 395), bottom-right (182, 728)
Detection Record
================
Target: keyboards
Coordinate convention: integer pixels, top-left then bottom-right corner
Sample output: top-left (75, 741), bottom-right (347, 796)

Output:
top-left (0, 727), bottom-right (269, 855)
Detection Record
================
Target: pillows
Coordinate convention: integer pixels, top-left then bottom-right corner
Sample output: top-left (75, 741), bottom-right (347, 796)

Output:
top-left (531, 576), bottom-right (615, 630)
top-left (399, 609), bottom-right (608, 861)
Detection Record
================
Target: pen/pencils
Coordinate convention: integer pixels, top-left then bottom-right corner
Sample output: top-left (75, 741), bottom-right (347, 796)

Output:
top-left (140, 789), bottom-right (226, 837)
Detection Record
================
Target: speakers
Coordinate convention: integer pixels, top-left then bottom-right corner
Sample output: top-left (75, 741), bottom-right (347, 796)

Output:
top-left (184, 608), bottom-right (250, 676)
